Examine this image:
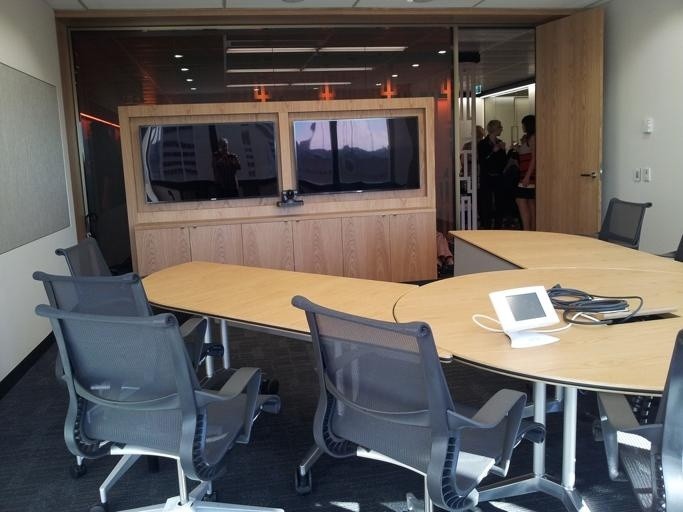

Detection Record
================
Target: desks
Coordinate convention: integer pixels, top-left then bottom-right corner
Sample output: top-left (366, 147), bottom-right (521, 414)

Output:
top-left (139, 261), bottom-right (454, 418)
top-left (393, 267), bottom-right (682, 512)
top-left (448, 230), bottom-right (683, 278)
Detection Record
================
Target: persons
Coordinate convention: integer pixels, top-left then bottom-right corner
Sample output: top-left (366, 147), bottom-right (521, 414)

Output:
top-left (436, 228), bottom-right (454, 278)
top-left (460, 115), bottom-right (535, 230)
top-left (212, 138), bottom-right (242, 198)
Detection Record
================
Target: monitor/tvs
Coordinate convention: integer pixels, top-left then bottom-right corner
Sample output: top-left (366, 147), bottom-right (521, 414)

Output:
top-left (489, 286), bottom-right (559, 334)
top-left (138, 121), bottom-right (279, 205)
top-left (293, 116), bottom-right (420, 196)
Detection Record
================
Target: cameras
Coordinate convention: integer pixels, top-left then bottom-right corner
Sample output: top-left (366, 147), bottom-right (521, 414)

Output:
top-left (228, 154), bottom-right (239, 160)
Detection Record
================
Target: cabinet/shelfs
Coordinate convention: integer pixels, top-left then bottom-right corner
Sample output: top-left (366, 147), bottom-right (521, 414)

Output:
top-left (241, 215), bottom-right (342, 275)
top-left (133, 217), bottom-right (242, 275)
top-left (341, 209), bottom-right (438, 283)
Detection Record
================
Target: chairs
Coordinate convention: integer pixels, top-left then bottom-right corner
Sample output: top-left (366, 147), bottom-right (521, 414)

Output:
top-left (657, 233), bottom-right (683, 263)
top-left (580, 198), bottom-right (655, 251)
top-left (595, 323), bottom-right (682, 511)
top-left (32, 303), bottom-right (286, 512)
top-left (55, 238), bottom-right (114, 277)
top-left (289, 293), bottom-right (547, 512)
top-left (31, 268), bottom-right (277, 476)
